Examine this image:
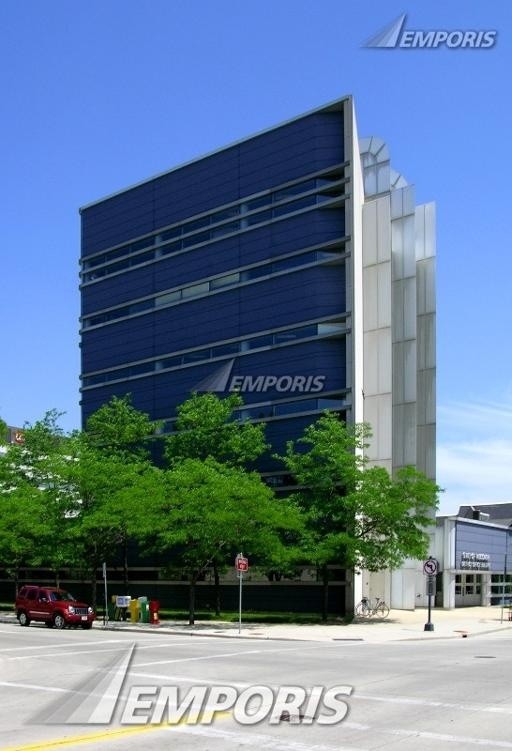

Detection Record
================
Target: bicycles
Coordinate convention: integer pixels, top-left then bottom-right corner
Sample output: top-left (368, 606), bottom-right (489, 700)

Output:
top-left (355, 596), bottom-right (392, 621)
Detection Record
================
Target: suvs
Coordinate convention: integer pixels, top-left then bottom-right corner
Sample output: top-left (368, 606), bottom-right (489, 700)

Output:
top-left (14, 583), bottom-right (97, 631)
